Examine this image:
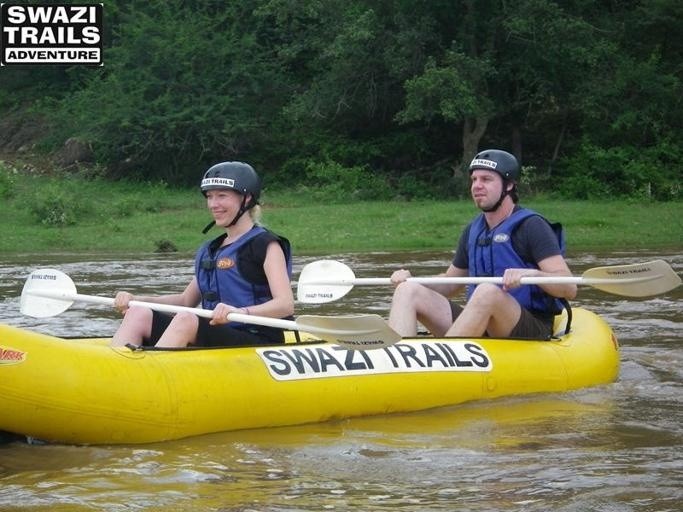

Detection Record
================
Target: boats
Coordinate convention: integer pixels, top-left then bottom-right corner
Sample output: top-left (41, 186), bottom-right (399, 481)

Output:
top-left (0, 299), bottom-right (623, 444)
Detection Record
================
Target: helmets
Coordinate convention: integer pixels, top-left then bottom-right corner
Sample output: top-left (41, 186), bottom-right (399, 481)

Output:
top-left (469, 149), bottom-right (521, 181)
top-left (200, 161), bottom-right (261, 200)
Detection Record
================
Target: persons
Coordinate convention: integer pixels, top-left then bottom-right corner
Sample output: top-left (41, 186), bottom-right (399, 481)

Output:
top-left (110, 162), bottom-right (294, 352)
top-left (387, 150), bottom-right (578, 340)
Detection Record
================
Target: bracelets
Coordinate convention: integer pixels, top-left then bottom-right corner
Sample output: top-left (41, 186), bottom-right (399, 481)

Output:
top-left (240, 307), bottom-right (249, 317)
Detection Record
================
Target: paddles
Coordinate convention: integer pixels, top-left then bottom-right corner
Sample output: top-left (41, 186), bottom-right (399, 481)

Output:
top-left (297, 260), bottom-right (683, 304)
top-left (20, 270), bottom-right (403, 350)
